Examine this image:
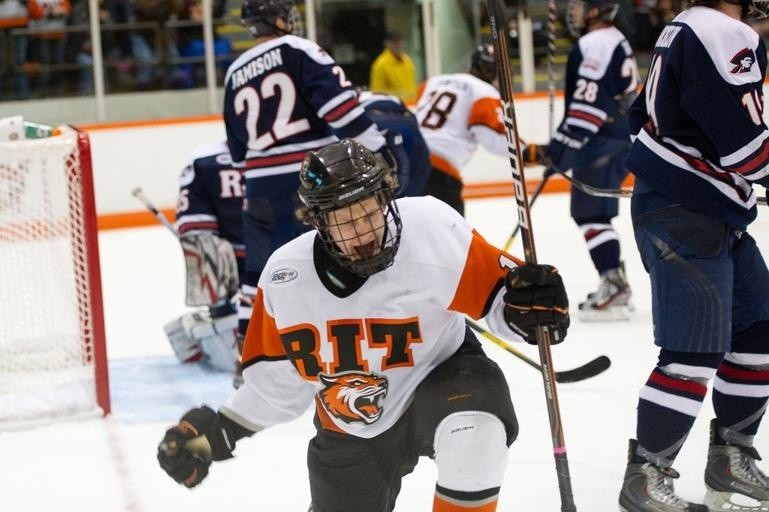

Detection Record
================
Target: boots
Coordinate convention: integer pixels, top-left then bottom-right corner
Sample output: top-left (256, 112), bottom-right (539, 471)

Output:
top-left (618, 435), bottom-right (713, 512)
top-left (705, 420), bottom-right (767, 499)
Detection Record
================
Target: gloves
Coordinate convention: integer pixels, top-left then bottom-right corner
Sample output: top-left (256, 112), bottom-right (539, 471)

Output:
top-left (546, 137), bottom-right (585, 173)
top-left (154, 401), bottom-right (233, 487)
top-left (501, 260), bottom-right (569, 345)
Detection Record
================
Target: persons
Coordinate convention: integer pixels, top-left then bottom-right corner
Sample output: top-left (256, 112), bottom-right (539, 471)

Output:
top-left (405, 42), bottom-right (549, 220)
top-left (356, 87), bottom-right (431, 197)
top-left (522, 0), bottom-right (642, 322)
top-left (1, 0), bottom-right (234, 99)
top-left (368, 31), bottom-right (417, 103)
top-left (616, 0), bottom-right (769, 512)
top-left (157, 136), bottom-right (570, 512)
top-left (612, 0), bottom-right (768, 56)
top-left (165, 0), bottom-right (403, 373)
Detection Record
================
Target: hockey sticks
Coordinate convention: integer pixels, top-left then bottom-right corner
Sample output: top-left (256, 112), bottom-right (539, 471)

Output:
top-left (522, 139), bottom-right (635, 200)
top-left (463, 319), bottom-right (611, 382)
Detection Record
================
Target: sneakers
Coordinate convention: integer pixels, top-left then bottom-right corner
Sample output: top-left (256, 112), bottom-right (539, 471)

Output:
top-left (578, 267), bottom-right (630, 310)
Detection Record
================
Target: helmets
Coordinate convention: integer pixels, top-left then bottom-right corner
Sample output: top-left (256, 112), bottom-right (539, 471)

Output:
top-left (568, 1), bottom-right (619, 35)
top-left (242, 1), bottom-right (300, 36)
top-left (467, 45), bottom-right (510, 88)
top-left (296, 139), bottom-right (402, 278)
